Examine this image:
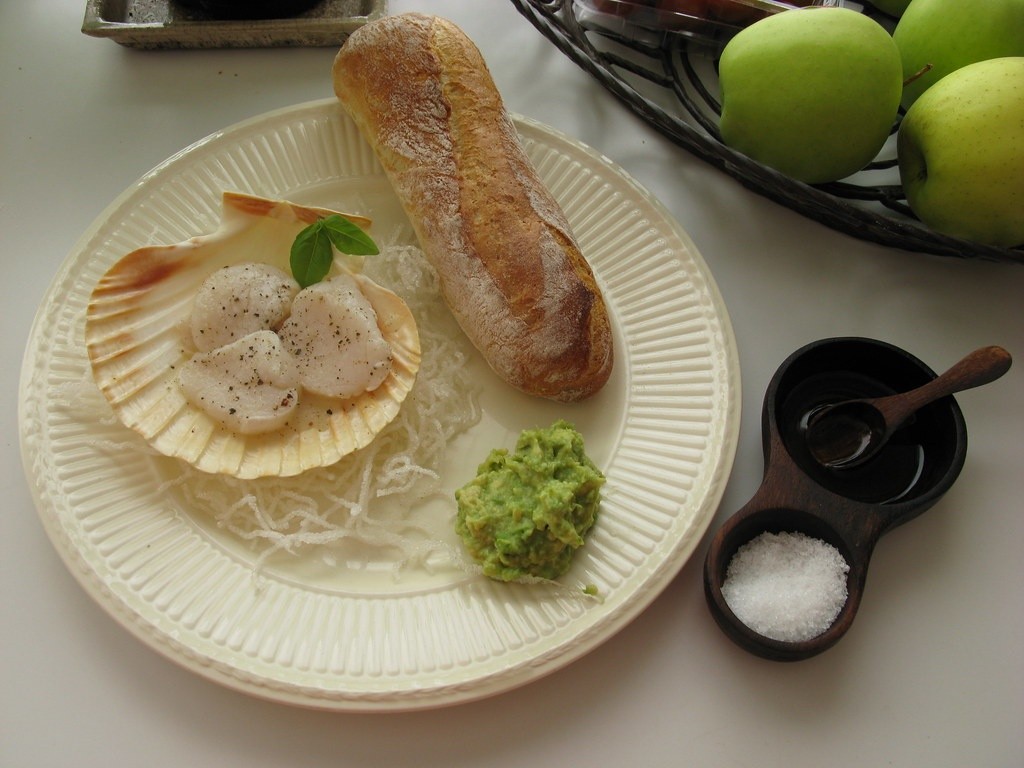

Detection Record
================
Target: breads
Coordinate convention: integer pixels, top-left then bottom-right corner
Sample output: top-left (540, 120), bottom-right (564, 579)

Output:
top-left (332, 13), bottom-right (615, 395)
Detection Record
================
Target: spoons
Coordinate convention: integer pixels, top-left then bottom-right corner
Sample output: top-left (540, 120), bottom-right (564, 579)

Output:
top-left (806, 344), bottom-right (1012, 467)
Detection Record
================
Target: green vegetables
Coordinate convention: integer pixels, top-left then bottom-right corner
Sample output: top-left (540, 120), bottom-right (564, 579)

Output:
top-left (289, 215), bottom-right (379, 289)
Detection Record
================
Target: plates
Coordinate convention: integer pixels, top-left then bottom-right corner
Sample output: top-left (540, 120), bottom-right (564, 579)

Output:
top-left (81, 1), bottom-right (389, 49)
top-left (19, 96), bottom-right (742, 713)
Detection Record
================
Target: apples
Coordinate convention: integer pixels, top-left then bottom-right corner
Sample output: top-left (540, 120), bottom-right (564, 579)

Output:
top-left (892, 0), bottom-right (1024, 127)
top-left (897, 55), bottom-right (1024, 251)
top-left (720, 4), bottom-right (902, 186)
